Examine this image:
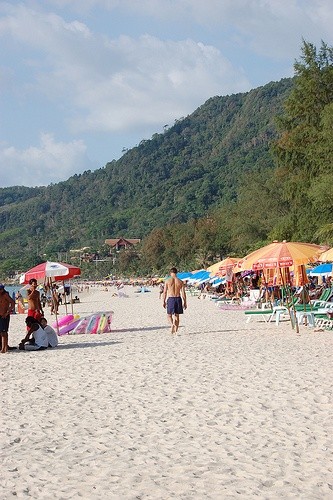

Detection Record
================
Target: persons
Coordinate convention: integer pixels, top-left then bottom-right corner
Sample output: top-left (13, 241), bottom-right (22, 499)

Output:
top-left (0, 278), bottom-right (58, 353)
top-left (162, 268), bottom-right (187, 334)
top-left (41, 285), bottom-right (60, 315)
top-left (58, 293), bottom-right (63, 305)
top-left (159, 285), bottom-right (164, 299)
top-left (105, 284), bottom-right (120, 297)
top-left (64, 296), bottom-right (79, 304)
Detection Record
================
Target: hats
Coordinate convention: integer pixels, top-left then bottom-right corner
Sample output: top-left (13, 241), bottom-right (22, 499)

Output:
top-left (0, 283), bottom-right (5, 288)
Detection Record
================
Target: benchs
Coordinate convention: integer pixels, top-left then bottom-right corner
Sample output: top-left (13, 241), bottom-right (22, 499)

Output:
top-left (212, 282), bottom-right (333, 333)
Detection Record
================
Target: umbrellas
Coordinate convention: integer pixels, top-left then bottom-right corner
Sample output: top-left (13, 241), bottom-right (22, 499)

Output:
top-left (88, 278), bottom-right (163, 284)
top-left (19, 260), bottom-right (81, 335)
top-left (19, 284), bottom-right (77, 292)
top-left (176, 240), bottom-right (333, 333)
